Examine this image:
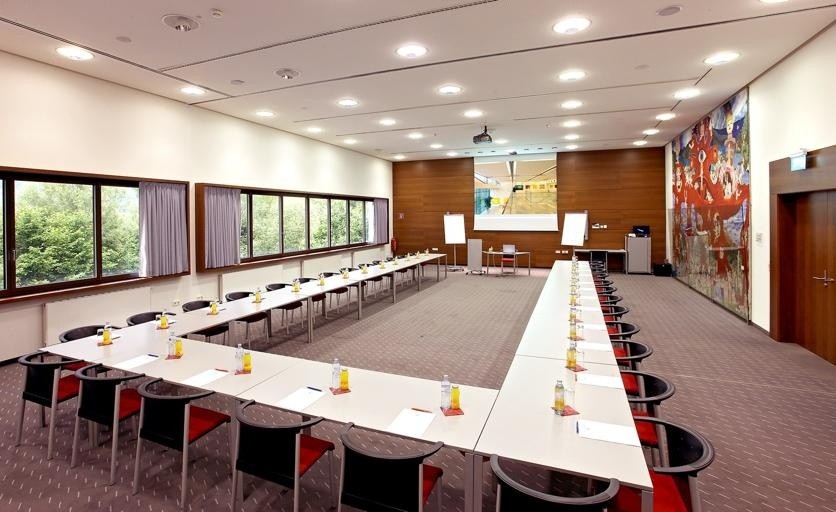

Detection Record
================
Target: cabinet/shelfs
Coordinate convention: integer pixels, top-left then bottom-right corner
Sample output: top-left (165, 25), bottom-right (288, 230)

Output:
top-left (623, 233), bottom-right (653, 275)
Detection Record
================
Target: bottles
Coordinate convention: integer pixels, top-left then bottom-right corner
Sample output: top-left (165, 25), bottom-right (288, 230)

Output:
top-left (175, 340), bottom-right (182, 357)
top-left (332, 358), bottom-right (341, 388)
top-left (439, 374), bottom-right (451, 412)
top-left (102, 247), bottom-right (430, 345)
top-left (242, 351), bottom-right (252, 372)
top-left (167, 331), bottom-right (176, 359)
top-left (554, 255), bottom-right (585, 415)
top-left (341, 367), bottom-right (349, 390)
top-left (452, 386), bottom-right (460, 410)
top-left (234, 343), bottom-right (244, 371)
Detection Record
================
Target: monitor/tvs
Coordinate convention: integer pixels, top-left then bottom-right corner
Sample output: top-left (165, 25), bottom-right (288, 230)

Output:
top-left (633, 225), bottom-right (650, 237)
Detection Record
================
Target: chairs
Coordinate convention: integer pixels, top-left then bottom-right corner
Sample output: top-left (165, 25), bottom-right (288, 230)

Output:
top-left (70, 361), bottom-right (146, 487)
top-left (483, 454), bottom-right (622, 512)
top-left (13, 350), bottom-right (94, 461)
top-left (43, 248), bottom-right (535, 374)
top-left (229, 398), bottom-right (336, 511)
top-left (334, 422), bottom-right (447, 512)
top-left (575, 253), bottom-right (682, 409)
top-left (129, 377), bottom-right (238, 509)
top-left (611, 413), bottom-right (716, 512)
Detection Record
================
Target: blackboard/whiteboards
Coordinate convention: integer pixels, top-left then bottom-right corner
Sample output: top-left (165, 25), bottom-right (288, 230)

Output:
top-left (443, 213), bottom-right (466, 245)
top-left (560, 213), bottom-right (587, 247)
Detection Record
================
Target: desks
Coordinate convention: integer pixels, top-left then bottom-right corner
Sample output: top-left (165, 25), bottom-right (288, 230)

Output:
top-left (35, 320), bottom-right (501, 509)
top-left (479, 248), bottom-right (532, 278)
top-left (474, 258), bottom-right (660, 511)
top-left (37, 249), bottom-right (465, 456)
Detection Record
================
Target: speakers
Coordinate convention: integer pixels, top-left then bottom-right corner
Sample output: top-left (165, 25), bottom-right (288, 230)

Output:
top-left (654, 264), bottom-right (671, 276)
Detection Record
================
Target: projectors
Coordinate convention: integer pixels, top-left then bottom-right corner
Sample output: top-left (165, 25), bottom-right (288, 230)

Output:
top-left (473, 134), bottom-right (492, 144)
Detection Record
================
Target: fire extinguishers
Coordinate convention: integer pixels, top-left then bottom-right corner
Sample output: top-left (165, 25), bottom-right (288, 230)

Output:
top-left (391, 238), bottom-right (397, 252)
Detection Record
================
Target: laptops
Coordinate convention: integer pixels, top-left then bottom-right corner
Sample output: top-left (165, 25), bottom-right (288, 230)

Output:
top-left (503, 245), bottom-right (515, 252)
